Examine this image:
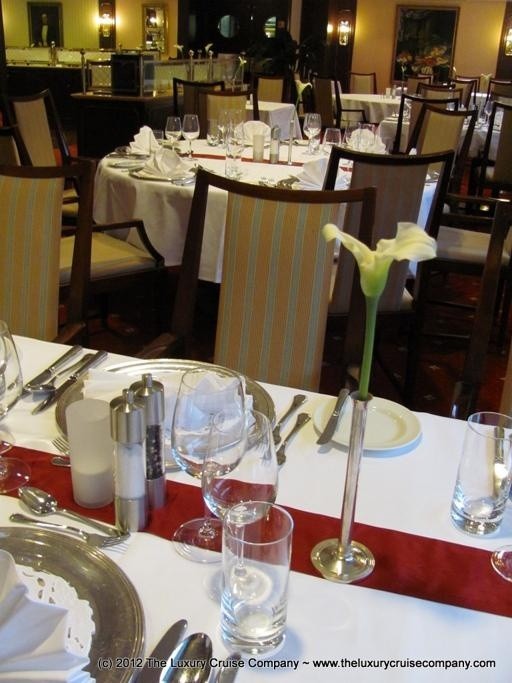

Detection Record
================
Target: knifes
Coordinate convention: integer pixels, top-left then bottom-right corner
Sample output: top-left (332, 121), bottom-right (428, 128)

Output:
top-left (17, 344), bottom-right (84, 397)
top-left (492, 424), bottom-right (506, 503)
top-left (315, 387), bottom-right (349, 447)
top-left (213, 655), bottom-right (241, 683)
top-left (33, 350), bottom-right (108, 415)
top-left (133, 619), bottom-right (190, 682)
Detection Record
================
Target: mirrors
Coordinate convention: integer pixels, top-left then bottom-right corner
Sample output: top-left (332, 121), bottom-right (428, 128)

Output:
top-left (141, 2), bottom-right (168, 55)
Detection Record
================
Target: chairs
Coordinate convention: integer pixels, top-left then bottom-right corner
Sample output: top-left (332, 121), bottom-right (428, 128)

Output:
top-left (373, 199), bottom-right (512, 424)
top-left (0, 95), bottom-right (166, 346)
top-left (323, 143), bottom-right (455, 395)
top-left (0, 163), bottom-right (87, 344)
top-left (153, 166), bottom-right (377, 396)
top-left (3, 73), bottom-right (512, 218)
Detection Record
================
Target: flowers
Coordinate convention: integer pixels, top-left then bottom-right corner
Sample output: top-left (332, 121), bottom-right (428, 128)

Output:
top-left (396, 52), bottom-right (413, 73)
top-left (410, 42), bottom-right (450, 74)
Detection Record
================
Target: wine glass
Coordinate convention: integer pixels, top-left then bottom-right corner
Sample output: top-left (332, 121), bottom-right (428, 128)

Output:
top-left (205, 406), bottom-right (273, 600)
top-left (1, 318), bottom-right (31, 495)
top-left (170, 363), bottom-right (247, 564)
top-left (154, 111), bottom-right (376, 191)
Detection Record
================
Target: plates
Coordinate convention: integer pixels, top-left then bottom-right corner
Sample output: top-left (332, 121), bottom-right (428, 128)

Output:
top-left (2, 526), bottom-right (145, 682)
top-left (313, 395), bottom-right (422, 450)
top-left (54, 357), bottom-right (272, 460)
top-left (423, 172), bottom-right (440, 183)
top-left (109, 137), bottom-right (196, 182)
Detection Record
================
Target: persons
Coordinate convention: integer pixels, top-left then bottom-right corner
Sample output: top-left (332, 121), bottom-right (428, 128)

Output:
top-left (277, 20), bottom-right (292, 41)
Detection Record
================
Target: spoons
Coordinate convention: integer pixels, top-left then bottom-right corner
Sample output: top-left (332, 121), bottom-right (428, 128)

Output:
top-left (160, 631), bottom-right (213, 683)
top-left (17, 485), bottom-right (133, 540)
top-left (26, 353), bottom-right (94, 394)
top-left (272, 393), bottom-right (308, 443)
top-left (274, 412), bottom-right (312, 466)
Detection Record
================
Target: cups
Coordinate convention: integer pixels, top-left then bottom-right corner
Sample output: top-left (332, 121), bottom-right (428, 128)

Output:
top-left (379, 83), bottom-right (397, 100)
top-left (452, 97), bottom-right (496, 129)
top-left (63, 398), bottom-right (117, 508)
top-left (448, 410), bottom-right (512, 537)
top-left (221, 504), bottom-right (293, 658)
top-left (156, 78), bottom-right (169, 91)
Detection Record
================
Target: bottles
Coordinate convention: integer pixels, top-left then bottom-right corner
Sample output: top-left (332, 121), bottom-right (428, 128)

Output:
top-left (110, 369), bottom-right (168, 531)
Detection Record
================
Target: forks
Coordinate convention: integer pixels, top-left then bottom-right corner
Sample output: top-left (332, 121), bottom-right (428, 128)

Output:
top-left (8, 512), bottom-right (131, 546)
top-left (51, 433), bottom-right (70, 457)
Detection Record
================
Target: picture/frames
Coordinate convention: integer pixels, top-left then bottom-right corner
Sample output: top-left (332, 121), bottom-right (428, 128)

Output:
top-left (27, 2), bottom-right (64, 47)
top-left (390, 4), bottom-right (460, 87)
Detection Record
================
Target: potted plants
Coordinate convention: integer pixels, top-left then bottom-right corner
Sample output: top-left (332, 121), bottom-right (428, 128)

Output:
top-left (309, 218), bottom-right (438, 584)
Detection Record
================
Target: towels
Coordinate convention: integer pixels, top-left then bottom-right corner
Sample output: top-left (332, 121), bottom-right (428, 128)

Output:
top-left (81, 367), bottom-right (256, 465)
top-left (2, 547), bottom-right (95, 682)
